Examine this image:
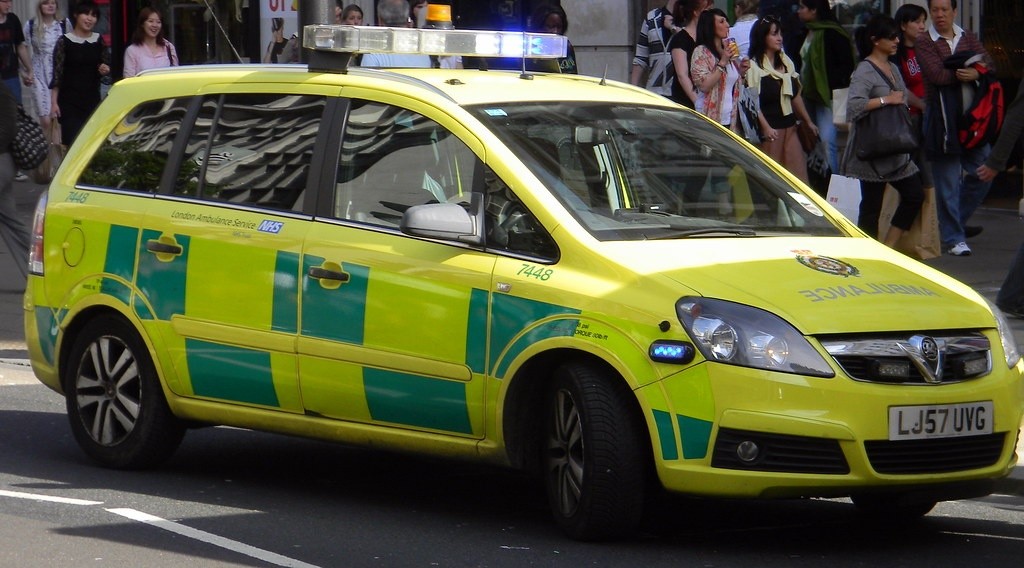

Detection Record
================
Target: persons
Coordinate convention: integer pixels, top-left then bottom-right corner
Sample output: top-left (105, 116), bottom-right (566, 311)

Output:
top-left (360, 0), bottom-right (432, 68)
top-left (49, 0), bottom-right (117, 156)
top-left (342, 4), bottom-right (363, 26)
top-left (846, 0), bottom-right (1024, 317)
top-left (410, 0), bottom-right (464, 69)
top-left (0, 0), bottom-right (35, 108)
top-left (630, 0), bottom-right (824, 228)
top-left (529, 0), bottom-right (578, 75)
top-left (0, 80), bottom-right (32, 282)
top-left (19, 0), bottom-right (74, 148)
top-left (122, 6), bottom-right (178, 79)
top-left (795, 0), bottom-right (857, 177)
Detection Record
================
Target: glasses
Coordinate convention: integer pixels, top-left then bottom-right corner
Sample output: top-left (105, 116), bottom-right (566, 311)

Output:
top-left (883, 32), bottom-right (901, 41)
top-left (760, 15), bottom-right (776, 26)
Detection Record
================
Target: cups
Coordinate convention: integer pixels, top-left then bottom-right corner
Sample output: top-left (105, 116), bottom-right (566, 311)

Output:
top-left (721, 38), bottom-right (739, 59)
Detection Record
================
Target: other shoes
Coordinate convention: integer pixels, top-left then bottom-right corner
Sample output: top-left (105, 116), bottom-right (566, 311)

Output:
top-left (996, 300), bottom-right (1024, 317)
top-left (964, 226), bottom-right (983, 238)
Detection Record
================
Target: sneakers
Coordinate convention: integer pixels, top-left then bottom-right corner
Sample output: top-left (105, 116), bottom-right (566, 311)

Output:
top-left (15, 171), bottom-right (28, 181)
top-left (947, 241), bottom-right (971, 255)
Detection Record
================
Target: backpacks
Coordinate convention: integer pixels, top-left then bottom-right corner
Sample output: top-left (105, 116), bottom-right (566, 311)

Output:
top-left (958, 63), bottom-right (1005, 153)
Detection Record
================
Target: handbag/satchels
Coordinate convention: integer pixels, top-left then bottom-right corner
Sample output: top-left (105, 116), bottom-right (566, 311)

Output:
top-left (9, 105), bottom-right (49, 170)
top-left (738, 85), bottom-right (762, 144)
top-left (826, 174), bottom-right (861, 227)
top-left (856, 60), bottom-right (917, 161)
top-left (35, 112), bottom-right (68, 184)
top-left (798, 121), bottom-right (817, 154)
top-left (877, 183), bottom-right (942, 259)
top-left (833, 87), bottom-right (850, 131)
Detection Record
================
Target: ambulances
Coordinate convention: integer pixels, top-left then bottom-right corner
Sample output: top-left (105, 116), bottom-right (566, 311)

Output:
top-left (21, 5), bottom-right (1023, 538)
